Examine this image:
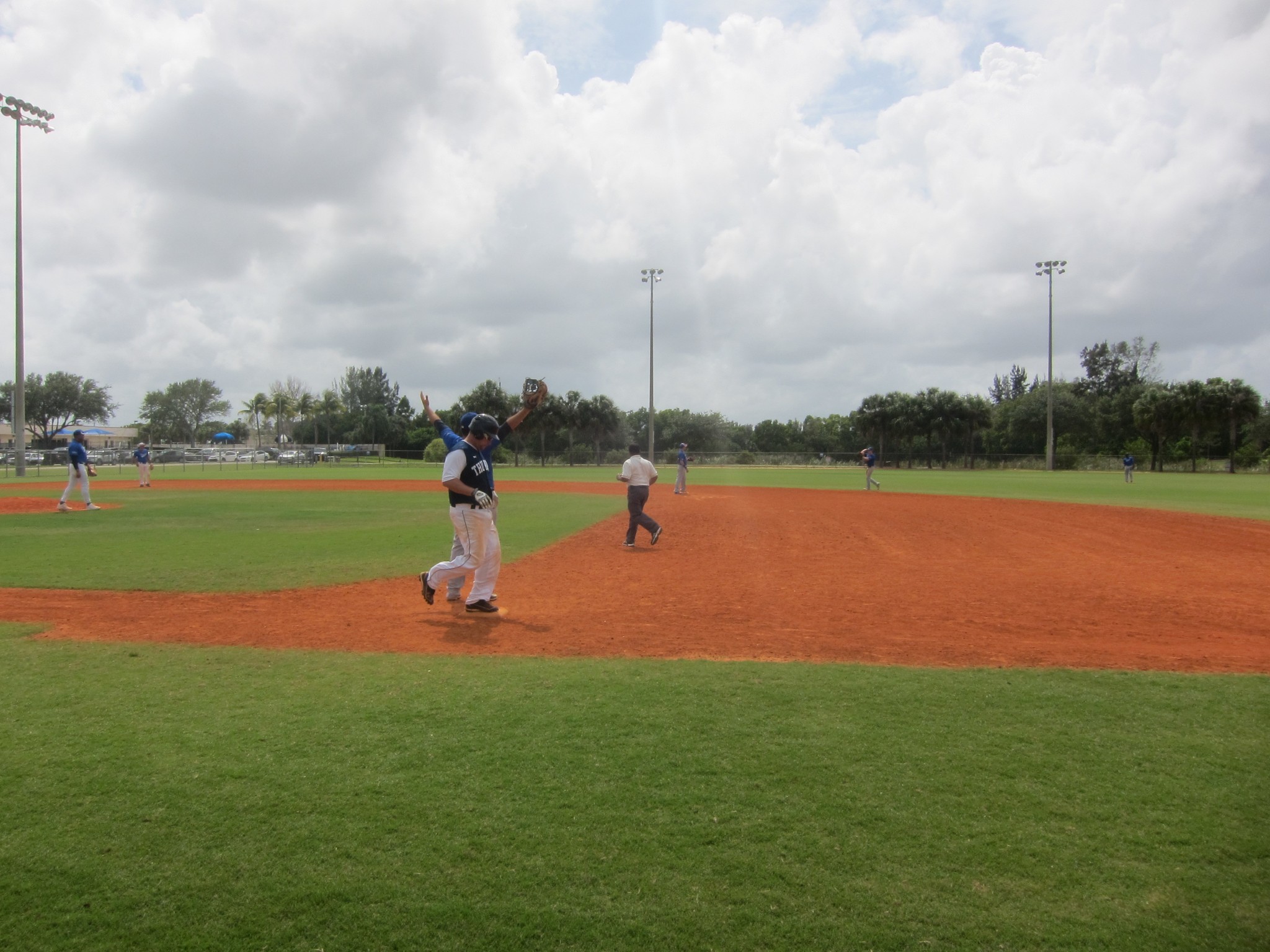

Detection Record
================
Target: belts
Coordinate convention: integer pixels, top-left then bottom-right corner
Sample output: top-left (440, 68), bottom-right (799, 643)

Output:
top-left (450, 504), bottom-right (483, 509)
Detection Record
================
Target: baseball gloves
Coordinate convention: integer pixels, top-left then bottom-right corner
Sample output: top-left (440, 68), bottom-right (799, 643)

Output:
top-left (522, 380), bottom-right (548, 409)
top-left (87, 469), bottom-right (98, 477)
top-left (150, 463), bottom-right (154, 470)
top-left (687, 457), bottom-right (694, 461)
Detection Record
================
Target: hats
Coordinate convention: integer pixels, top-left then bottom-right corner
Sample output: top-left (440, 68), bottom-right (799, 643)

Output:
top-left (1125, 453), bottom-right (1129, 454)
top-left (139, 443), bottom-right (144, 446)
top-left (73, 430), bottom-right (88, 436)
top-left (868, 448), bottom-right (872, 451)
top-left (680, 443), bottom-right (687, 448)
top-left (461, 412), bottom-right (477, 427)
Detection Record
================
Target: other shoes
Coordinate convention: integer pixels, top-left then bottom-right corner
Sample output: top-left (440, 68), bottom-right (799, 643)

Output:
top-left (1125, 481), bottom-right (1128, 483)
top-left (1131, 480), bottom-right (1133, 483)
top-left (86, 503), bottom-right (100, 510)
top-left (680, 491), bottom-right (687, 493)
top-left (877, 482), bottom-right (880, 491)
top-left (674, 492), bottom-right (682, 494)
top-left (446, 590), bottom-right (461, 600)
top-left (140, 484), bottom-right (144, 487)
top-left (146, 484), bottom-right (150, 487)
top-left (489, 594), bottom-right (497, 601)
top-left (863, 488), bottom-right (870, 490)
top-left (57, 503), bottom-right (72, 510)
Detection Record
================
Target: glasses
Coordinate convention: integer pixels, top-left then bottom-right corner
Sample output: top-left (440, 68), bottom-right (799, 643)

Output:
top-left (482, 433), bottom-right (492, 441)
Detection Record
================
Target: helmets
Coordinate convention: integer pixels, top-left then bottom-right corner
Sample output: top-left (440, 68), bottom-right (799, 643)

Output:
top-left (468, 414), bottom-right (500, 441)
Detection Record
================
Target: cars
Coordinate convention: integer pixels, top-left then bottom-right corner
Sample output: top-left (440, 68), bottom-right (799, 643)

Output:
top-left (258, 448), bottom-right (281, 461)
top-left (239, 450), bottom-right (270, 463)
top-left (153, 448), bottom-right (194, 463)
top-left (207, 451), bottom-right (240, 463)
top-left (108, 447), bottom-right (157, 465)
top-left (44, 446), bottom-right (71, 465)
top-left (346, 445), bottom-right (363, 451)
top-left (0, 447), bottom-right (44, 466)
top-left (278, 450), bottom-right (305, 465)
top-left (86, 449), bottom-right (119, 467)
top-left (186, 447), bottom-right (220, 462)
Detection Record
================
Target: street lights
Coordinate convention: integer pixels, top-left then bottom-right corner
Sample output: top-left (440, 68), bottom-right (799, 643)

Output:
top-left (0, 95), bottom-right (56, 478)
top-left (1035, 258), bottom-right (1067, 471)
top-left (640, 266), bottom-right (665, 465)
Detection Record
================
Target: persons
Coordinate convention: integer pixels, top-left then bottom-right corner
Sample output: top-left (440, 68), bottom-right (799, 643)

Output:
top-left (1122, 453), bottom-right (1135, 483)
top-left (56, 430), bottom-right (101, 511)
top-left (616, 444), bottom-right (662, 547)
top-left (418, 379), bottom-right (547, 612)
top-left (861, 447), bottom-right (880, 490)
top-left (133, 443), bottom-right (151, 487)
top-left (673, 443), bottom-right (695, 494)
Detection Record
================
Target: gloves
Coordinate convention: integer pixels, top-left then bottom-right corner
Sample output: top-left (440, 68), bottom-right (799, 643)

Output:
top-left (472, 488), bottom-right (492, 508)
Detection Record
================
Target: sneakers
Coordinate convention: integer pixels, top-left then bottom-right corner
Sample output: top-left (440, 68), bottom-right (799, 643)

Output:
top-left (651, 527), bottom-right (662, 545)
top-left (418, 572), bottom-right (435, 605)
top-left (465, 599), bottom-right (498, 612)
top-left (622, 541), bottom-right (634, 546)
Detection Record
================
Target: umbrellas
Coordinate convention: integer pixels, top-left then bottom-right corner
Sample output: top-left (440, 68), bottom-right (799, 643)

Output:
top-left (214, 432), bottom-right (234, 440)
top-left (38, 428), bottom-right (75, 435)
top-left (274, 434), bottom-right (292, 443)
top-left (82, 429), bottom-right (117, 435)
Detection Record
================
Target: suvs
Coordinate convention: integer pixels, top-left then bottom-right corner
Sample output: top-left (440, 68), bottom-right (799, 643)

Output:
top-left (312, 447), bottom-right (329, 461)
